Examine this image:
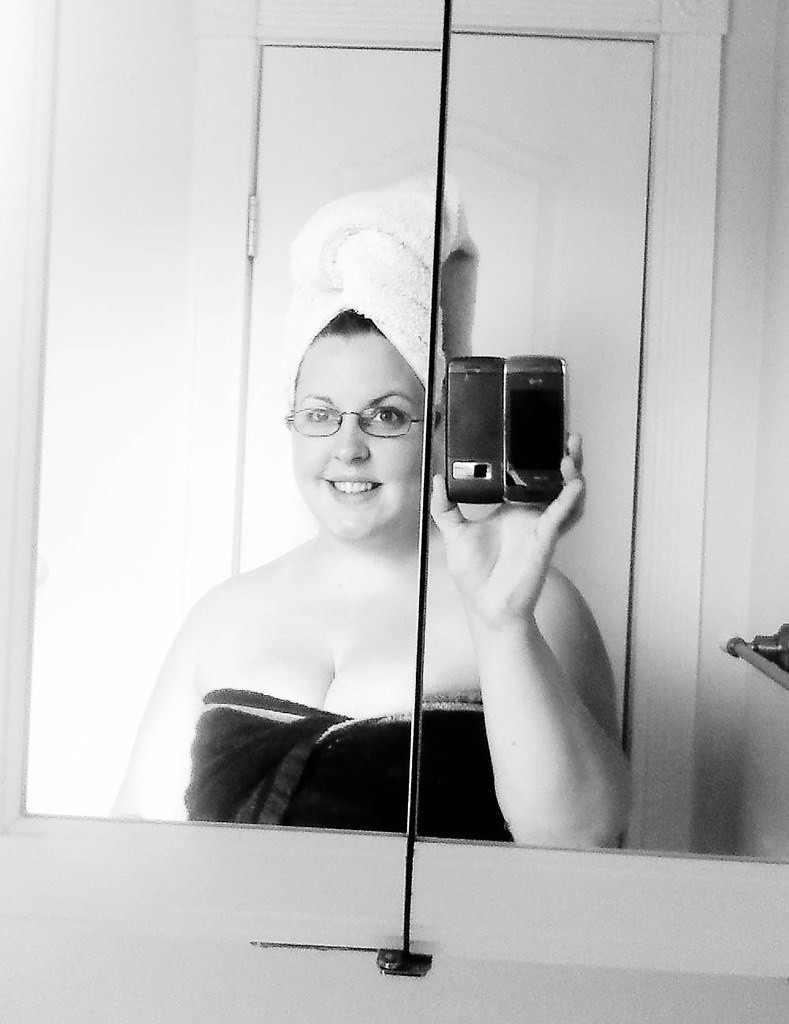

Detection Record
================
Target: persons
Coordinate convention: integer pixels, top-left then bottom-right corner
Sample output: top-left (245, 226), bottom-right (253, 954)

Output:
top-left (106, 172), bottom-right (636, 849)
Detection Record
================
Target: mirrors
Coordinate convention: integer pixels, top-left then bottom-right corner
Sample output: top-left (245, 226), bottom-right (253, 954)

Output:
top-left (19, 0), bottom-right (789, 867)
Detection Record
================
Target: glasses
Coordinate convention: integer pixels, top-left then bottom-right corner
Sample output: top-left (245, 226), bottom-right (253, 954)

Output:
top-left (285, 402), bottom-right (422, 438)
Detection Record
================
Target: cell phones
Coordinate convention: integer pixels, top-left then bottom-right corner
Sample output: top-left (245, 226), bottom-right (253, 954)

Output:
top-left (444, 355), bottom-right (567, 506)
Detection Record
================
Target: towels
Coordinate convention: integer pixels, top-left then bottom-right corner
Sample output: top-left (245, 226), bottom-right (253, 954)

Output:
top-left (286, 173), bottom-right (479, 411)
top-left (187, 686), bottom-right (516, 848)
